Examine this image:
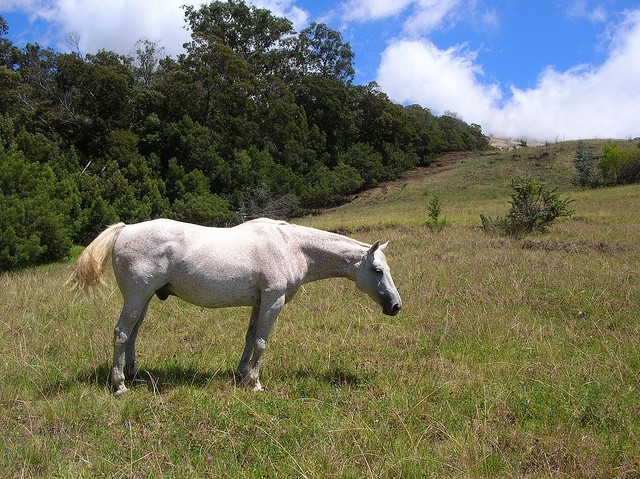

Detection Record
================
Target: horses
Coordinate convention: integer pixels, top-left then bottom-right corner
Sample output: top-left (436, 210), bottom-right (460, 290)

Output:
top-left (61, 216), bottom-right (403, 397)
